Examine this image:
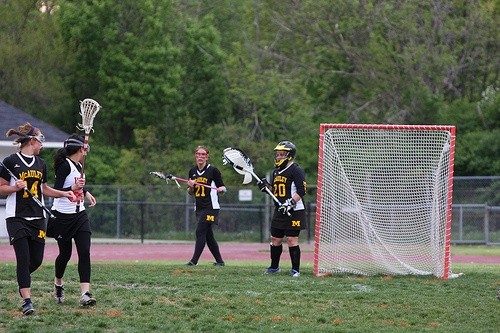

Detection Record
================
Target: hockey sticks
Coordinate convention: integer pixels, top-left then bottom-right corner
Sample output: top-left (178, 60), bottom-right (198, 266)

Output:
top-left (0, 160), bottom-right (57, 220)
top-left (223, 147), bottom-right (293, 219)
top-left (74, 98), bottom-right (100, 213)
top-left (148, 170), bottom-right (227, 192)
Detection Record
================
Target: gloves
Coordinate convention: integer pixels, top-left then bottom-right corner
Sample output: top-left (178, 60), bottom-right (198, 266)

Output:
top-left (278, 198), bottom-right (296, 214)
top-left (258, 177), bottom-right (274, 193)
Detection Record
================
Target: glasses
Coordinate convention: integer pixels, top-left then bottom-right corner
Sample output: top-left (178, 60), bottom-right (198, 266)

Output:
top-left (194, 152), bottom-right (208, 159)
top-left (64, 138), bottom-right (90, 152)
top-left (19, 133), bottom-right (45, 144)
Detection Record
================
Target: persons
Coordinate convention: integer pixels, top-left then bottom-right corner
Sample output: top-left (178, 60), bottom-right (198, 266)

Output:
top-left (256, 141), bottom-right (308, 278)
top-left (182, 145), bottom-right (226, 266)
top-left (44, 133), bottom-right (96, 305)
top-left (0, 122), bottom-right (76, 316)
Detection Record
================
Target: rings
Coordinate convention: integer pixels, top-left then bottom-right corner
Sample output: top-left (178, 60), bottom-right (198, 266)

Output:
top-left (18, 180), bottom-right (20, 183)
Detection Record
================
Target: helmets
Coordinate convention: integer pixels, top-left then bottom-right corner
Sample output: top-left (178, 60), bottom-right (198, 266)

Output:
top-left (274, 141), bottom-right (297, 168)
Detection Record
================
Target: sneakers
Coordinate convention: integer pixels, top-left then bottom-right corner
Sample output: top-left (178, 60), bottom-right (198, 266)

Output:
top-left (262, 266), bottom-right (281, 275)
top-left (78, 290), bottom-right (97, 306)
top-left (54, 278), bottom-right (65, 303)
top-left (289, 269), bottom-right (301, 277)
top-left (22, 299), bottom-right (34, 314)
